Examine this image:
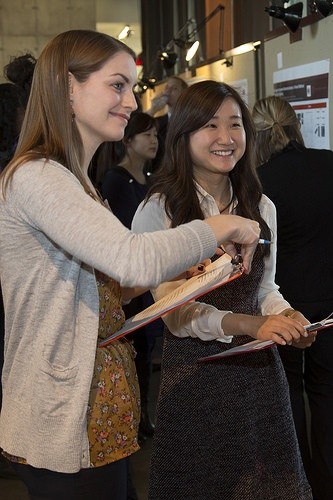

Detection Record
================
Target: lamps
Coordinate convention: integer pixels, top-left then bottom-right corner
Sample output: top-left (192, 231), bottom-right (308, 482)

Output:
top-left (264, 0), bottom-right (303, 33)
top-left (138, 80), bottom-right (149, 94)
top-left (174, 5), bottom-right (232, 67)
top-left (158, 19), bottom-right (196, 77)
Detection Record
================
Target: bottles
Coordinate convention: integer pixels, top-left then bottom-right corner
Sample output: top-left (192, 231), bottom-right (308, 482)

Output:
top-left (153, 95), bottom-right (171, 108)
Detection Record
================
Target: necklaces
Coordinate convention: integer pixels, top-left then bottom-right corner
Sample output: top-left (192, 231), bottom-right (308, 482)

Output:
top-left (218, 185), bottom-right (235, 213)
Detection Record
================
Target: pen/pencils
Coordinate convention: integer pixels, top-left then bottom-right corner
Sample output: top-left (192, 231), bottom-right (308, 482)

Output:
top-left (258, 237), bottom-right (275, 246)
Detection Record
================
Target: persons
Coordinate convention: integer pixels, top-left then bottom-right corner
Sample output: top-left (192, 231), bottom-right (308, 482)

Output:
top-left (0, 30), bottom-right (261, 500)
top-left (0, 54), bottom-right (38, 175)
top-left (102, 113), bottom-right (159, 232)
top-left (146, 76), bottom-right (188, 138)
top-left (250, 96), bottom-right (333, 500)
top-left (131, 78), bottom-right (317, 500)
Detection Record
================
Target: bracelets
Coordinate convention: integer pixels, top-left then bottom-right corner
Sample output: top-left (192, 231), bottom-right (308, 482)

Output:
top-left (284, 310), bottom-right (296, 317)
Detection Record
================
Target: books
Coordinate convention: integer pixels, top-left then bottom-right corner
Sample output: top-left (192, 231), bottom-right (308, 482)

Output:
top-left (198, 313), bottom-right (333, 361)
